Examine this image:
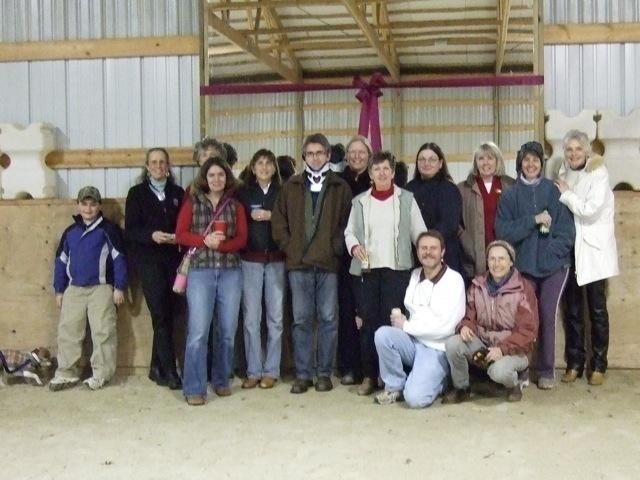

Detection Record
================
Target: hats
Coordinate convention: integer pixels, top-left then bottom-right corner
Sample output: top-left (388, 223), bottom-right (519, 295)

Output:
top-left (519, 142), bottom-right (544, 160)
top-left (485, 239), bottom-right (515, 267)
top-left (78, 186), bottom-right (101, 202)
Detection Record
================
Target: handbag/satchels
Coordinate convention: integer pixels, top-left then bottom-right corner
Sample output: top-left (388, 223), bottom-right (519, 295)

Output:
top-left (172, 250), bottom-right (193, 296)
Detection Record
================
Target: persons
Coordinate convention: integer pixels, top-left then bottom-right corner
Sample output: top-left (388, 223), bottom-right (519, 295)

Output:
top-left (127, 128), bottom-right (619, 410)
top-left (48, 186), bottom-right (128, 390)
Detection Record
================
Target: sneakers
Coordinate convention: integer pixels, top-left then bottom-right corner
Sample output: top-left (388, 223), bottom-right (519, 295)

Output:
top-left (537, 373), bottom-right (556, 388)
top-left (442, 385), bottom-right (471, 405)
top-left (504, 380), bottom-right (524, 402)
top-left (82, 375), bottom-right (109, 391)
top-left (48, 376), bottom-right (81, 391)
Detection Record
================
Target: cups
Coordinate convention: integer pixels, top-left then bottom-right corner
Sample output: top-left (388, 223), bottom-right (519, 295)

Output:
top-left (214, 220), bottom-right (226, 235)
top-left (251, 204), bottom-right (263, 211)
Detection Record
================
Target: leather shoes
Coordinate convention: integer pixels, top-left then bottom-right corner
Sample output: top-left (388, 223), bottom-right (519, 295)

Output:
top-left (214, 385), bottom-right (232, 397)
top-left (242, 365), bottom-right (404, 405)
top-left (182, 391), bottom-right (205, 406)
top-left (588, 371), bottom-right (607, 385)
top-left (561, 369), bottom-right (583, 382)
top-left (148, 361), bottom-right (183, 390)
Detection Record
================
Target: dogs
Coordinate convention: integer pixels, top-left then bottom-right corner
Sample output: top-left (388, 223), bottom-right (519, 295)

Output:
top-left (0, 346), bottom-right (54, 387)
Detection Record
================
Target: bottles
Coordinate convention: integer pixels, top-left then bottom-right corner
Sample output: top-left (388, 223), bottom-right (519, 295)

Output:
top-left (537, 205), bottom-right (552, 239)
top-left (361, 247), bottom-right (371, 274)
